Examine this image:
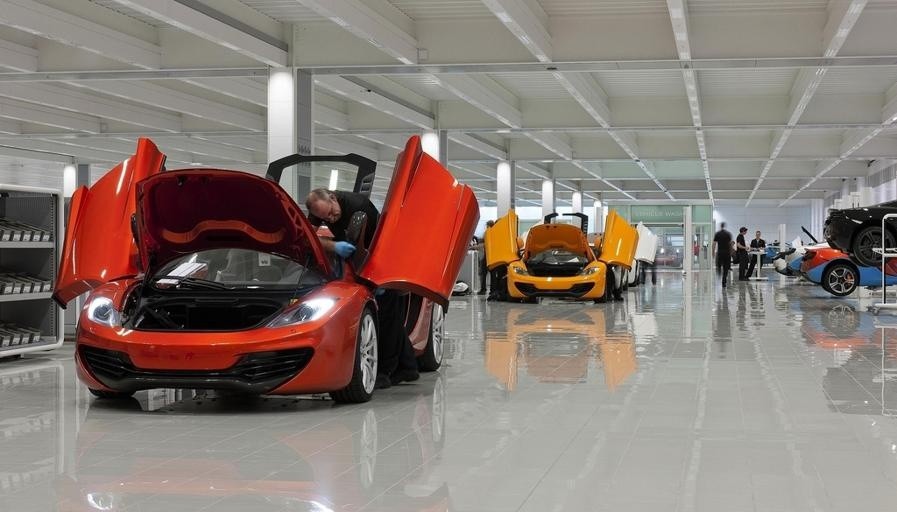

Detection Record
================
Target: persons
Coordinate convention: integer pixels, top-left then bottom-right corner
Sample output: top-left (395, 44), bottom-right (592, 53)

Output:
top-left (745, 230), bottom-right (765, 282)
top-left (712, 222), bottom-right (733, 288)
top-left (305, 187), bottom-right (420, 389)
top-left (735, 226), bottom-right (749, 281)
top-left (468, 219), bottom-right (496, 294)
top-left (729, 241), bottom-right (735, 270)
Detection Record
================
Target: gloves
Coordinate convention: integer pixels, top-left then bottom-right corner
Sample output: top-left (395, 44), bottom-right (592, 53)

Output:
top-left (335, 241), bottom-right (356, 260)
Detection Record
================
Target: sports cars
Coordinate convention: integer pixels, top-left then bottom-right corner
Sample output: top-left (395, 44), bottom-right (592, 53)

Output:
top-left (484, 208), bottom-right (661, 304)
top-left (55, 134), bottom-right (481, 404)
top-left (747, 241), bottom-right (793, 264)
top-left (772, 198), bottom-right (897, 296)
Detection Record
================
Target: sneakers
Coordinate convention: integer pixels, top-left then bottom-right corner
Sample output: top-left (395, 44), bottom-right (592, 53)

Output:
top-left (375, 363), bottom-right (421, 390)
top-left (477, 290), bottom-right (486, 295)
